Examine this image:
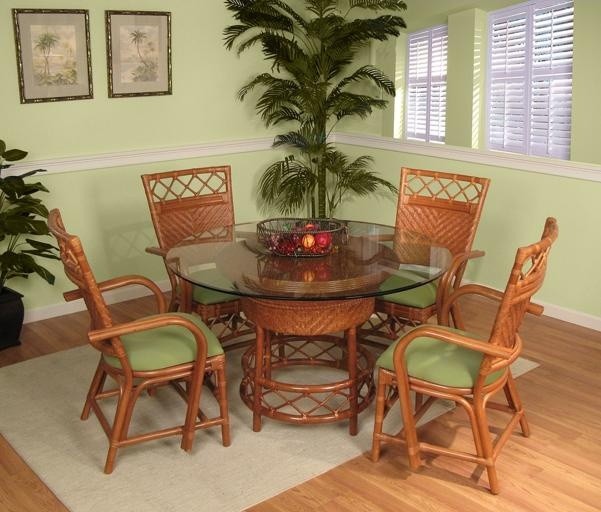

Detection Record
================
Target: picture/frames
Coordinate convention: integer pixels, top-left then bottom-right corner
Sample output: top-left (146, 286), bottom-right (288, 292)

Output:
top-left (11, 8), bottom-right (94, 105)
top-left (104, 9), bottom-right (172, 98)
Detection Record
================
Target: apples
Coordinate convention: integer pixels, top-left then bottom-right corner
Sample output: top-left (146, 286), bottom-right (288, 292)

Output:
top-left (302, 234), bottom-right (315, 248)
top-left (315, 230), bottom-right (332, 248)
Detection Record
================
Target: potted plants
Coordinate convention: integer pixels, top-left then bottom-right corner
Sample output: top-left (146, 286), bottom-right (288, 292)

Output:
top-left (0, 138), bottom-right (61, 351)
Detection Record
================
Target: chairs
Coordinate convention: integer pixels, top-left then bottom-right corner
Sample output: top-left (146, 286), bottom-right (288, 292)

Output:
top-left (47, 208), bottom-right (230, 475)
top-left (139, 164), bottom-right (285, 362)
top-left (342, 166), bottom-right (491, 362)
top-left (371, 217), bottom-right (560, 496)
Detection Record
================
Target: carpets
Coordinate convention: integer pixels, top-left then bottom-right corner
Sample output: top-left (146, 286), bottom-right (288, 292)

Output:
top-left (0, 311), bottom-right (541, 512)
